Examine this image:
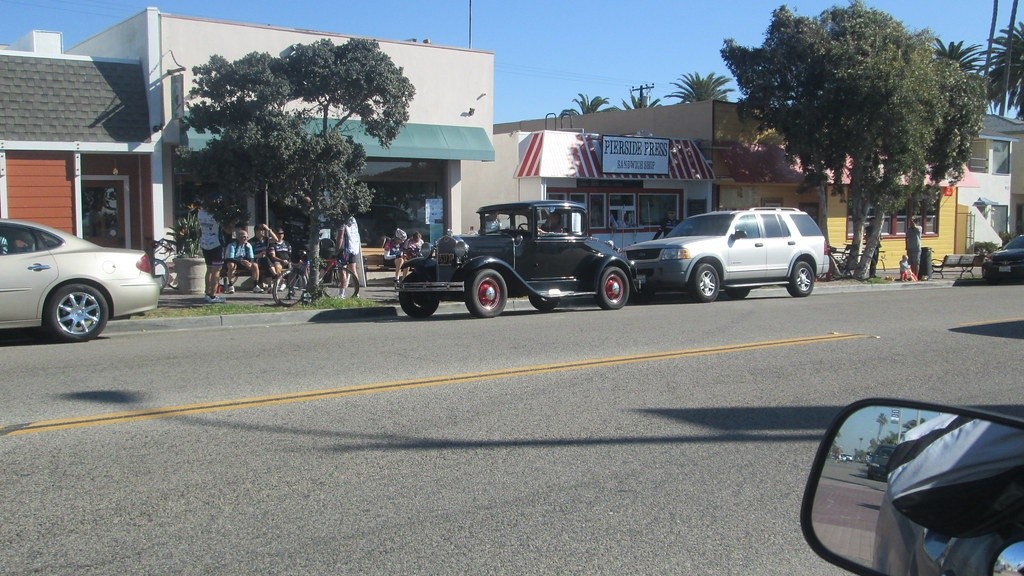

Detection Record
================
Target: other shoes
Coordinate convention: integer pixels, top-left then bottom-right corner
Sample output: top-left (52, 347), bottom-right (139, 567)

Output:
top-left (205, 295), bottom-right (226, 303)
top-left (268, 286), bottom-right (273, 293)
top-left (354, 293), bottom-right (359, 298)
top-left (253, 285), bottom-right (264, 293)
top-left (224, 286), bottom-right (235, 294)
top-left (392, 279), bottom-right (401, 288)
top-left (271, 273), bottom-right (279, 280)
top-left (333, 289), bottom-right (345, 299)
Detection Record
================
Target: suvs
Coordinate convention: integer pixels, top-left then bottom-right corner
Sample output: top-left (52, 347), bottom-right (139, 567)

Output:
top-left (620, 205), bottom-right (832, 305)
top-left (393, 200), bottom-right (642, 320)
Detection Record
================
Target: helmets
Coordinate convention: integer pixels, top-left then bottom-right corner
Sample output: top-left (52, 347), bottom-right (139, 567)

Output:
top-left (395, 228), bottom-right (407, 242)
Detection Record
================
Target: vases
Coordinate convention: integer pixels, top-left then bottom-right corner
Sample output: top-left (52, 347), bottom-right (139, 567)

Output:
top-left (172, 258), bottom-right (207, 295)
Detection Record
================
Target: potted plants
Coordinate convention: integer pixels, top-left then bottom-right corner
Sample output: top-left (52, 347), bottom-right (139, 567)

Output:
top-left (975, 242), bottom-right (997, 267)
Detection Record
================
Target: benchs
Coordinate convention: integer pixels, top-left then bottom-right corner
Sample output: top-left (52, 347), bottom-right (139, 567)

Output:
top-left (931, 254), bottom-right (978, 280)
top-left (220, 268), bottom-right (299, 293)
top-left (361, 249), bottom-right (435, 287)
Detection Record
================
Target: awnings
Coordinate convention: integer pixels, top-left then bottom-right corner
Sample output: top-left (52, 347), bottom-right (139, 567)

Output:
top-left (719, 141), bottom-right (981, 188)
top-left (179, 110), bottom-right (496, 163)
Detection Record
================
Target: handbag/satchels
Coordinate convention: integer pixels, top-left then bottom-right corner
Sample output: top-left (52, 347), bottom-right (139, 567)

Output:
top-left (336, 249), bottom-right (347, 265)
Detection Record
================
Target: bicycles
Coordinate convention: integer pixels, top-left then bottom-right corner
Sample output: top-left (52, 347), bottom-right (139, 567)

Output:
top-left (272, 238), bottom-right (360, 307)
top-left (828, 243), bottom-right (887, 278)
top-left (142, 238), bottom-right (177, 292)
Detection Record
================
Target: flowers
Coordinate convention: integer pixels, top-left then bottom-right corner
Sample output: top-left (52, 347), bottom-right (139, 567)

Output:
top-left (163, 204), bottom-right (202, 258)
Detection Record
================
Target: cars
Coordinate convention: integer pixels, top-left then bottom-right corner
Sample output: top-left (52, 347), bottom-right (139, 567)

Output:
top-left (981, 234), bottom-right (1024, 286)
top-left (864, 443), bottom-right (897, 482)
top-left (0, 219), bottom-right (161, 342)
top-left (800, 396), bottom-right (1023, 576)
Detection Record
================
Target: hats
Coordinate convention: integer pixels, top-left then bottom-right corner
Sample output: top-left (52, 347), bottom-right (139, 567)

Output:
top-left (275, 227), bottom-right (284, 233)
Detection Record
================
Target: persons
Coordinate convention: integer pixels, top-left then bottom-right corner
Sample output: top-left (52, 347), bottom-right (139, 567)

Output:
top-left (653, 208), bottom-right (681, 241)
top-left (382, 228), bottom-right (425, 283)
top-left (864, 218), bottom-right (882, 277)
top-left (900, 217), bottom-right (922, 282)
top-left (478, 211), bottom-right (501, 235)
top-left (537, 213), bottom-right (564, 235)
top-left (198, 196), bottom-right (360, 304)
top-left (1, 228), bottom-right (36, 255)
